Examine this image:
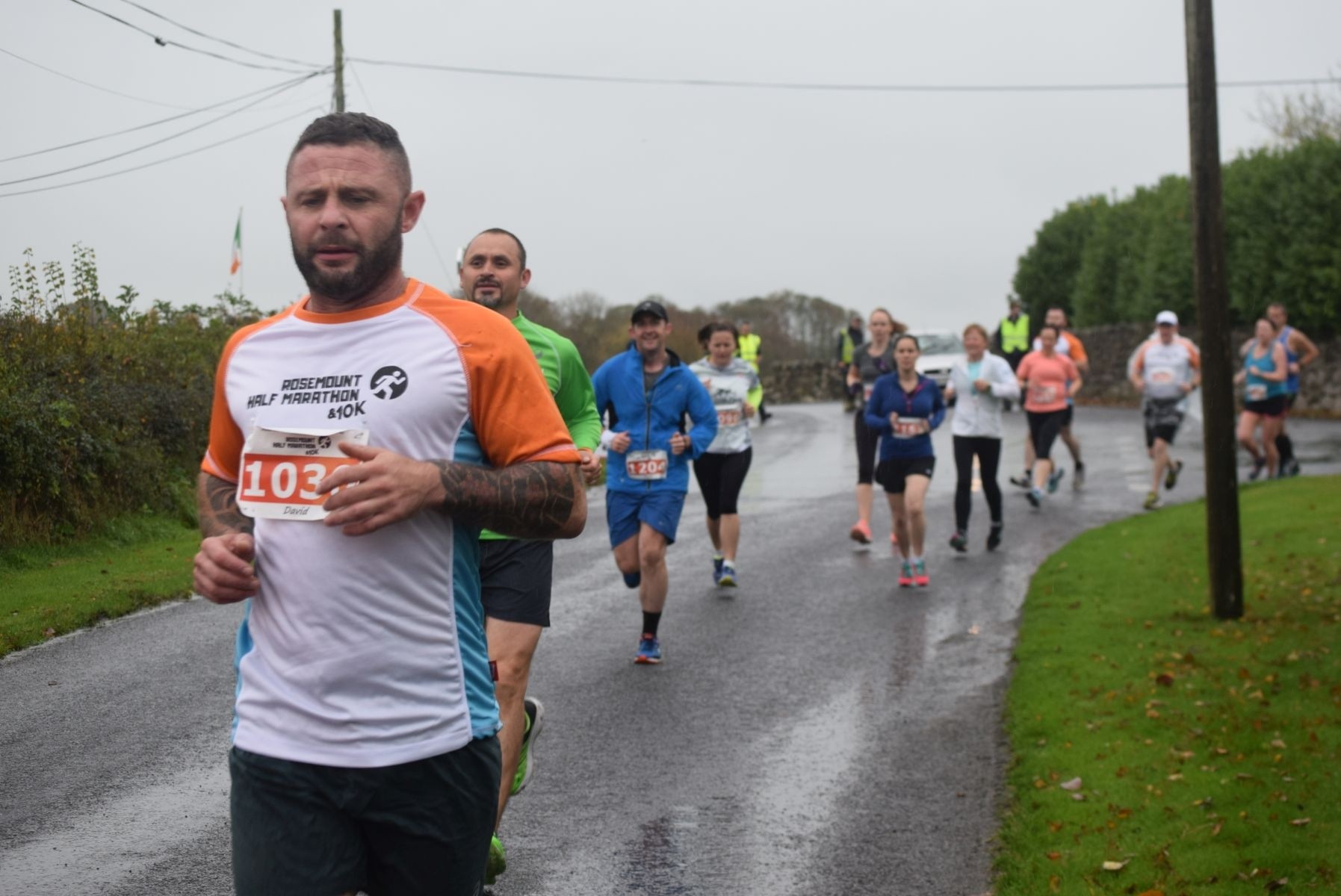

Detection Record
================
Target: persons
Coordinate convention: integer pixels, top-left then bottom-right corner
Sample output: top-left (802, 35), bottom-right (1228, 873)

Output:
top-left (1232, 300), bottom-right (1321, 480)
top-left (847, 307), bottom-right (909, 545)
top-left (943, 323), bottom-right (1020, 554)
top-left (1009, 305), bottom-right (1091, 494)
top-left (456, 229), bottom-right (605, 887)
top-left (737, 320), bottom-right (774, 426)
top-left (1014, 323), bottom-right (1083, 510)
top-left (865, 335), bottom-right (946, 587)
top-left (994, 299), bottom-right (1034, 415)
top-left (591, 300), bottom-right (719, 666)
top-left (688, 318), bottom-right (764, 590)
top-left (1128, 310), bottom-right (1201, 509)
top-left (835, 310), bottom-right (865, 412)
top-left (188, 111), bottom-right (587, 896)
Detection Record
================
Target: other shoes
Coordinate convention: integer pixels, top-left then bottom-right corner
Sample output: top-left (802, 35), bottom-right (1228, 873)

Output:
top-left (1145, 491), bottom-right (1158, 508)
top-left (1009, 474), bottom-right (1030, 487)
top-left (1074, 461), bottom-right (1085, 484)
top-left (1249, 456), bottom-right (1268, 478)
top-left (1165, 461), bottom-right (1184, 487)
top-left (1277, 461), bottom-right (1300, 477)
top-left (1047, 468), bottom-right (1063, 492)
top-left (1025, 490), bottom-right (1041, 506)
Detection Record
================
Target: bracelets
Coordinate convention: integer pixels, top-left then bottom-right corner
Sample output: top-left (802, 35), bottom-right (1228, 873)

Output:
top-left (1298, 362), bottom-right (1304, 370)
top-left (1191, 382), bottom-right (1197, 391)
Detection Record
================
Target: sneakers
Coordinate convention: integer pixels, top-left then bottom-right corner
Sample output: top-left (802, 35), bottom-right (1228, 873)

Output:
top-left (910, 558), bottom-right (928, 584)
top-left (898, 558), bottom-right (912, 585)
top-left (890, 533), bottom-right (899, 544)
top-left (950, 534), bottom-right (966, 550)
top-left (510, 694), bottom-right (544, 793)
top-left (985, 522), bottom-right (1001, 548)
top-left (485, 831), bottom-right (506, 884)
top-left (850, 520), bottom-right (872, 543)
top-left (719, 565), bottom-right (737, 586)
top-left (712, 556), bottom-right (723, 585)
top-left (622, 570), bottom-right (642, 589)
top-left (634, 635), bottom-right (662, 663)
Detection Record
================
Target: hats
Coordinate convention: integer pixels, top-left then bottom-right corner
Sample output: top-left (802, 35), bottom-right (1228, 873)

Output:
top-left (1156, 310), bottom-right (1177, 326)
top-left (631, 300), bottom-right (668, 322)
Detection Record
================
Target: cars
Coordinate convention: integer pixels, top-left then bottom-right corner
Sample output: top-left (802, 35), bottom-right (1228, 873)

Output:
top-left (914, 334), bottom-right (967, 406)
